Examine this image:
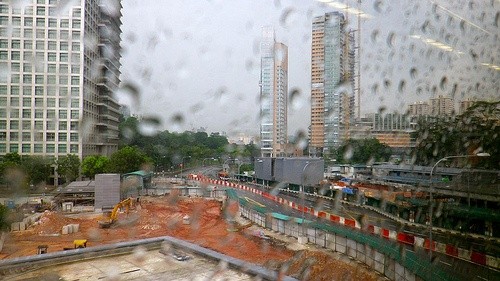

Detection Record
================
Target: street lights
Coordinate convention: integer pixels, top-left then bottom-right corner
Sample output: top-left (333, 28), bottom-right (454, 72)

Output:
top-left (222, 158), bottom-right (239, 171)
top-left (201, 156), bottom-right (215, 173)
top-left (180, 155), bottom-right (191, 178)
top-left (427, 152), bottom-right (491, 262)
top-left (300, 157), bottom-right (338, 235)
top-left (235, 159), bottom-right (263, 196)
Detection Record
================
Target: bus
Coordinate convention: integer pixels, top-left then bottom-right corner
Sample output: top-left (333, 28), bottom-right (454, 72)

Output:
top-left (218, 172), bottom-right (230, 181)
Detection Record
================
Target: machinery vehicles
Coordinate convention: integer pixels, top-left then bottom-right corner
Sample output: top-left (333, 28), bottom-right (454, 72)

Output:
top-left (96, 197), bottom-right (132, 229)
top-left (63, 238), bottom-right (87, 250)
top-left (37, 244), bottom-right (48, 254)
